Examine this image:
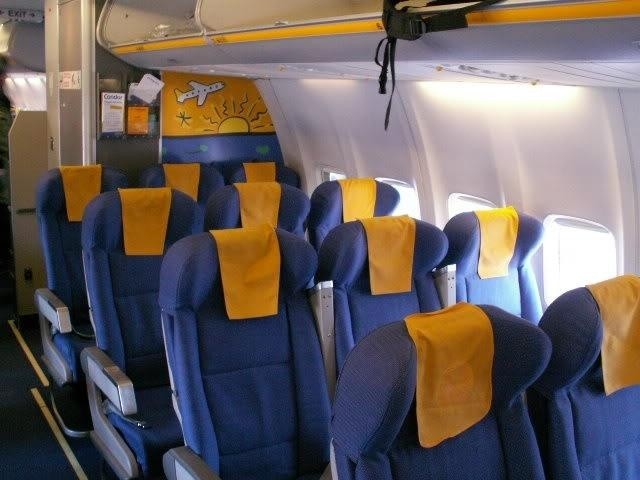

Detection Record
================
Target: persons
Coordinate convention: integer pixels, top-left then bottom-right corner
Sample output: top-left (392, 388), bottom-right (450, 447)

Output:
top-left (0, 55), bottom-right (13, 209)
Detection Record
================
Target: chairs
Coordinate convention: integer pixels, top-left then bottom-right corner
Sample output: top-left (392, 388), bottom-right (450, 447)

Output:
top-left (523, 274), bottom-right (640, 480)
top-left (329, 300), bottom-right (556, 480)
top-left (307, 176), bottom-right (402, 254)
top-left (137, 162), bottom-right (224, 202)
top-left (158, 226), bottom-right (327, 479)
top-left (225, 160), bottom-right (302, 190)
top-left (318, 215), bottom-right (450, 405)
top-left (444, 205), bottom-right (546, 328)
top-left (206, 181), bottom-right (312, 233)
top-left (80, 186), bottom-right (204, 479)
top-left (33, 164), bottom-right (136, 439)
